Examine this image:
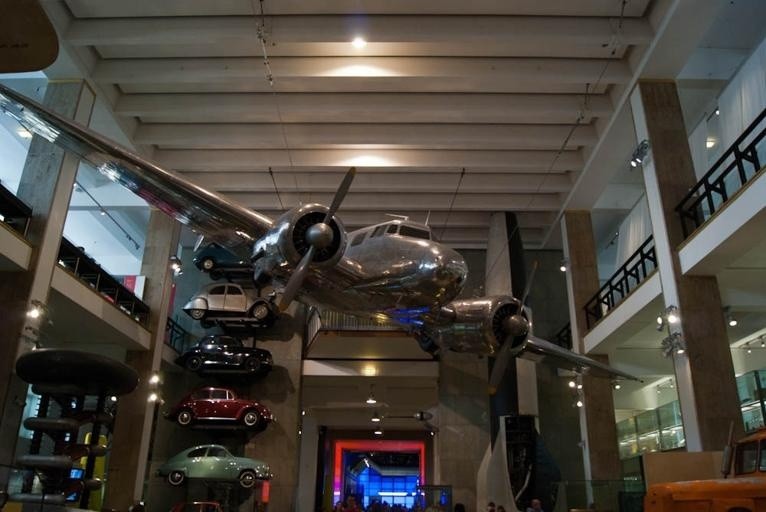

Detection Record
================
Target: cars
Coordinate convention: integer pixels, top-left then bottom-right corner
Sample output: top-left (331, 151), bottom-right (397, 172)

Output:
top-left (193, 233), bottom-right (251, 270)
top-left (173, 334), bottom-right (273, 378)
top-left (165, 501), bottom-right (221, 512)
top-left (164, 386), bottom-right (275, 434)
top-left (153, 444), bottom-right (273, 489)
top-left (180, 281), bottom-right (277, 330)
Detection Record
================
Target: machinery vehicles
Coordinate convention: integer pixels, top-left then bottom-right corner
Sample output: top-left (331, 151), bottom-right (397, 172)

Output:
top-left (643, 420), bottom-right (765, 512)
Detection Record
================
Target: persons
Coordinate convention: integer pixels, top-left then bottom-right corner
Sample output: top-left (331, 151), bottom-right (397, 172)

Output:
top-left (334, 494), bottom-right (544, 512)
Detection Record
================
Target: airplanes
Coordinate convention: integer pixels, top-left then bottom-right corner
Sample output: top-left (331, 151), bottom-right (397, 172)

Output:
top-left (0, 80), bottom-right (649, 395)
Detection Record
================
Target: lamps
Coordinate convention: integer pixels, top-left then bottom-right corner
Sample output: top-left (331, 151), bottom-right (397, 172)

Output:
top-left (654, 304), bottom-right (678, 333)
top-left (658, 331), bottom-right (686, 358)
top-left (363, 380), bottom-right (384, 436)
top-left (722, 305), bottom-right (738, 328)
top-left (629, 138), bottom-right (651, 170)
